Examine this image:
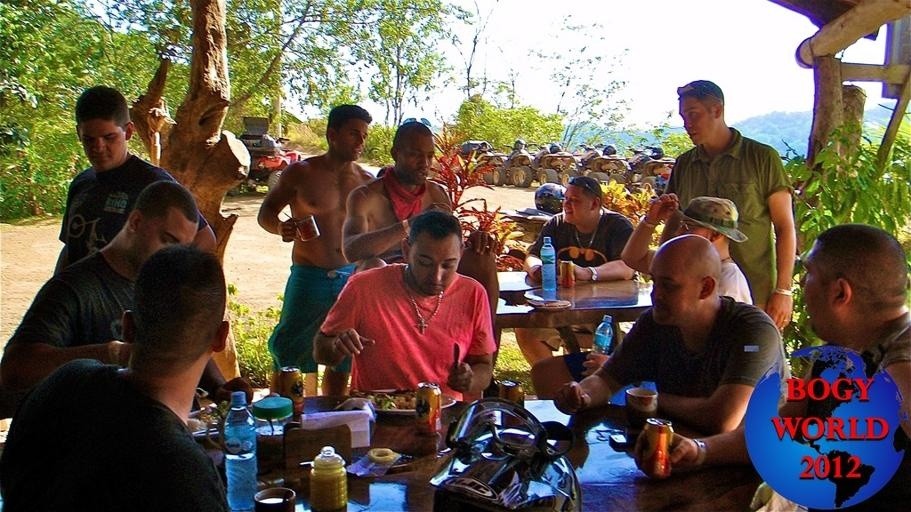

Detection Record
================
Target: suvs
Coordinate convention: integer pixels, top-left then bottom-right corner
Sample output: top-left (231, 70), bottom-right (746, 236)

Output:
top-left (453, 140), bottom-right (676, 192)
top-left (226, 116), bottom-right (301, 196)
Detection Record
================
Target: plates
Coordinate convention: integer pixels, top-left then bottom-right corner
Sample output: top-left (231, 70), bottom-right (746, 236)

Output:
top-left (536, 301), bottom-right (572, 309)
top-left (360, 388), bottom-right (455, 413)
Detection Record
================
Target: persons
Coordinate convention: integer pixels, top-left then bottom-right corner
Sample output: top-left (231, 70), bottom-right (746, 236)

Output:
top-left (632, 222), bottom-right (911, 511)
top-left (0, 179), bottom-right (255, 422)
top-left (551, 233), bottom-right (786, 437)
top-left (54, 83), bottom-right (220, 276)
top-left (664, 79), bottom-right (799, 332)
top-left (531, 191), bottom-right (755, 399)
top-left (513, 175), bottom-right (640, 367)
top-left (253, 103), bottom-right (382, 399)
top-left (312, 207), bottom-right (499, 403)
top-left (1, 243), bottom-right (230, 511)
top-left (340, 121), bottom-right (504, 397)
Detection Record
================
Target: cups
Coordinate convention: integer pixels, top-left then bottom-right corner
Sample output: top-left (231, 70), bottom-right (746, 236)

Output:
top-left (254, 485), bottom-right (298, 511)
top-left (295, 214), bottom-right (321, 243)
top-left (627, 386), bottom-right (658, 438)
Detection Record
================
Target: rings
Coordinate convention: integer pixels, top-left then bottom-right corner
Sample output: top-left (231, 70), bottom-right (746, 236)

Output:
top-left (784, 318), bottom-right (789, 322)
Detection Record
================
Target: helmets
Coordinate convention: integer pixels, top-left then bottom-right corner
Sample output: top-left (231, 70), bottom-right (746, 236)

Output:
top-left (430, 428), bottom-right (581, 512)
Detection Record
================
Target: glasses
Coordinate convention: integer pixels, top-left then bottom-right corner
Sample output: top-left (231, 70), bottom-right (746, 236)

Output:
top-left (568, 177), bottom-right (601, 197)
top-left (445, 397), bottom-right (578, 462)
top-left (679, 220), bottom-right (705, 231)
top-left (403, 118), bottom-right (432, 126)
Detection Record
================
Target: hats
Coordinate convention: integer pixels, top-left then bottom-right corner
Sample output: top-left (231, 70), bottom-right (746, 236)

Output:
top-left (676, 196), bottom-right (748, 243)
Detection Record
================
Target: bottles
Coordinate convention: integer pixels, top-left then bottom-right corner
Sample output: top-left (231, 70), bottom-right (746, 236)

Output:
top-left (592, 313), bottom-right (616, 357)
top-left (540, 235), bottom-right (558, 291)
top-left (308, 445), bottom-right (350, 510)
top-left (252, 397), bottom-right (294, 472)
top-left (227, 391), bottom-right (258, 510)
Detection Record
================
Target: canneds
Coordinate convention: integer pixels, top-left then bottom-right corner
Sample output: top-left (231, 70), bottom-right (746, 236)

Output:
top-left (416, 382), bottom-right (441, 436)
top-left (560, 260), bottom-right (575, 288)
top-left (500, 380), bottom-right (516, 404)
top-left (643, 418), bottom-right (673, 479)
top-left (279, 366), bottom-right (304, 414)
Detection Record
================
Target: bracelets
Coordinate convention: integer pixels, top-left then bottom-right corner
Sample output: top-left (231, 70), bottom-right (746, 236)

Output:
top-left (585, 265), bottom-right (599, 283)
top-left (402, 218), bottom-right (412, 237)
top-left (691, 437), bottom-right (708, 467)
top-left (775, 287), bottom-right (794, 296)
top-left (641, 216), bottom-right (657, 229)
top-left (109, 339), bottom-right (125, 365)
top-left (528, 262), bottom-right (543, 279)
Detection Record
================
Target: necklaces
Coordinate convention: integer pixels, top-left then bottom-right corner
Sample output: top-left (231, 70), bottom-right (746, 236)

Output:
top-left (575, 209), bottom-right (603, 250)
top-left (404, 265), bottom-right (445, 334)
